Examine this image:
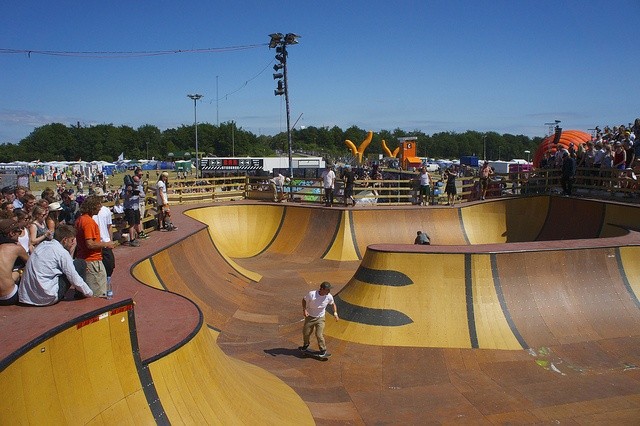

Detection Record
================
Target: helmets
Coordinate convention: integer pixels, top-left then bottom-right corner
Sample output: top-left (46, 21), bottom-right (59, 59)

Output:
top-left (320, 282), bottom-right (334, 289)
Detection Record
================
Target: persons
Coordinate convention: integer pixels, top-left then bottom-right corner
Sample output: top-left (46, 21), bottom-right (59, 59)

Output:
top-left (47, 201), bottom-right (63, 232)
top-left (300, 280), bottom-right (340, 357)
top-left (499, 177), bottom-right (513, 196)
top-left (538, 123), bottom-right (640, 195)
top-left (442, 165), bottom-right (458, 206)
top-left (341, 166), bottom-right (355, 208)
top-left (122, 175), bottom-right (141, 247)
top-left (167, 168), bottom-right (247, 195)
top-left (0, 166), bottom-right (127, 212)
top-left (480, 161), bottom-right (493, 201)
top-left (0, 219), bottom-right (30, 304)
top-left (317, 164), bottom-right (337, 206)
top-left (403, 175), bottom-right (420, 204)
top-left (418, 166), bottom-right (434, 206)
top-left (414, 229), bottom-right (431, 245)
top-left (18, 225), bottom-right (94, 305)
top-left (14, 211), bottom-right (30, 255)
top-left (512, 181), bottom-right (518, 195)
top-left (30, 205), bottom-right (52, 243)
top-left (153, 172), bottom-right (178, 232)
top-left (488, 166), bottom-right (495, 181)
top-left (266, 172), bottom-right (288, 205)
top-left (364, 160), bottom-right (387, 186)
top-left (76, 196), bottom-right (108, 298)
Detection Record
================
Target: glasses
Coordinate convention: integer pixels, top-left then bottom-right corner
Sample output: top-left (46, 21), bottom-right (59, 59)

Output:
top-left (36, 212), bottom-right (48, 217)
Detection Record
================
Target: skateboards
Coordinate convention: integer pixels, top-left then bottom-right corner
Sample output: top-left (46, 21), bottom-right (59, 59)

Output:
top-left (298, 346), bottom-right (333, 361)
top-left (162, 205), bottom-right (174, 232)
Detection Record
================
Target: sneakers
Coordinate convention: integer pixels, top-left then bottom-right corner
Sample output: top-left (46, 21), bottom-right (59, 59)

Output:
top-left (319, 350), bottom-right (327, 357)
top-left (127, 241), bottom-right (140, 246)
top-left (137, 234), bottom-right (150, 238)
top-left (49, 202), bottom-right (62, 212)
top-left (301, 345), bottom-right (308, 352)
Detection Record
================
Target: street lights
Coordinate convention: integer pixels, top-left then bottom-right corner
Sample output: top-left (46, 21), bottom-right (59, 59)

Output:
top-left (269, 32), bottom-right (301, 202)
top-left (524, 150), bottom-right (531, 173)
top-left (187, 93), bottom-right (204, 178)
top-left (482, 135), bottom-right (488, 160)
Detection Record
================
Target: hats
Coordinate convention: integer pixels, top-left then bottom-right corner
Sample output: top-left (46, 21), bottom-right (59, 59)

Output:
top-left (0, 219), bottom-right (19, 234)
